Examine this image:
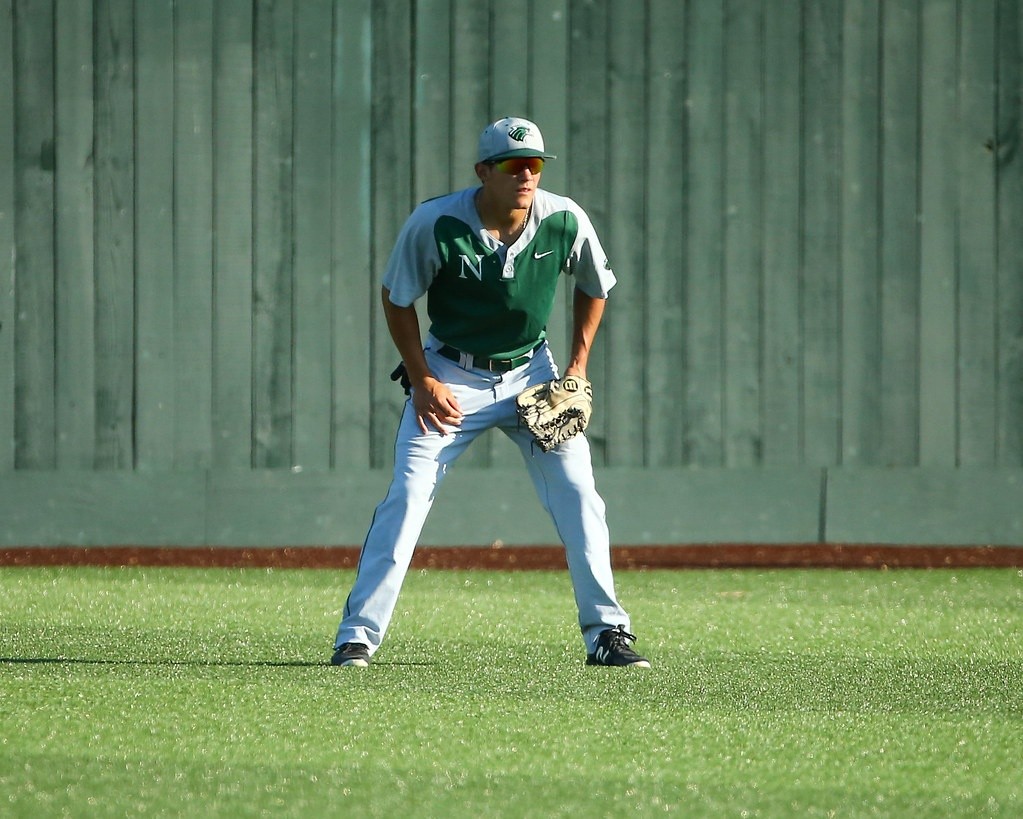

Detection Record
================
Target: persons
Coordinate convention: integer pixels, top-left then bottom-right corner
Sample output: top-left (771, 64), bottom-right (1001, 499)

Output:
top-left (330, 117), bottom-right (649, 667)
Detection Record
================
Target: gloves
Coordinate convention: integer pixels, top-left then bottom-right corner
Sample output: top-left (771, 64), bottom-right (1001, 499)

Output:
top-left (391, 360), bottom-right (411, 396)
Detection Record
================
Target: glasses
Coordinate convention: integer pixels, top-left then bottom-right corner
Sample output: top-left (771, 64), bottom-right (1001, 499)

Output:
top-left (495, 159), bottom-right (544, 175)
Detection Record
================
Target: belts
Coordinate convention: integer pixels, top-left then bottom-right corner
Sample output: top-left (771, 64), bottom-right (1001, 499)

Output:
top-left (436, 339), bottom-right (547, 372)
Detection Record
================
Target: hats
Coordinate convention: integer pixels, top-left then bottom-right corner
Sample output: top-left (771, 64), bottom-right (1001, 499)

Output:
top-left (478, 117), bottom-right (557, 163)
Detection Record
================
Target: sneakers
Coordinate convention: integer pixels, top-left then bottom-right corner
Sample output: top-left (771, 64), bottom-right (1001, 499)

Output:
top-left (330, 643), bottom-right (371, 667)
top-left (585, 624), bottom-right (651, 668)
